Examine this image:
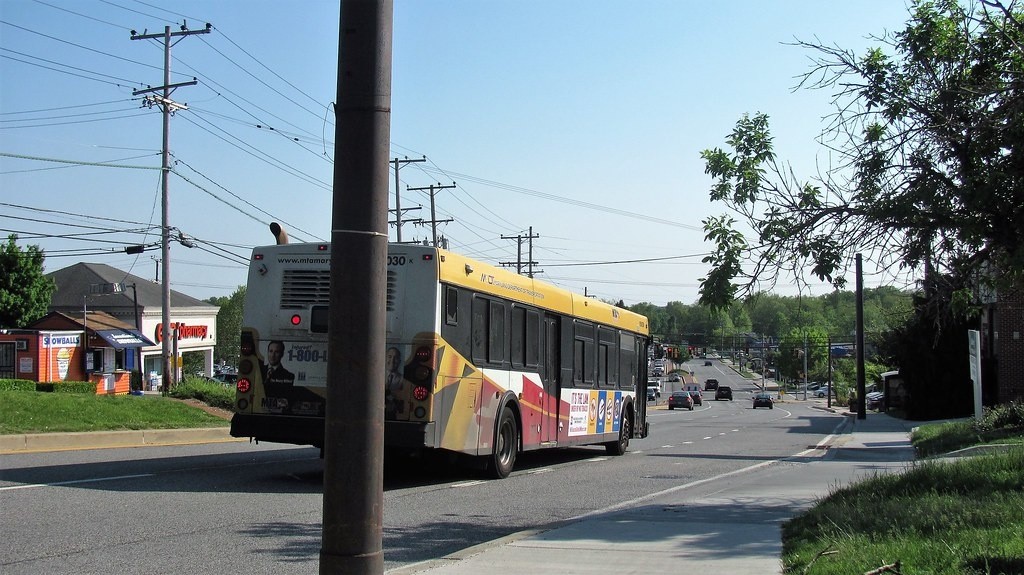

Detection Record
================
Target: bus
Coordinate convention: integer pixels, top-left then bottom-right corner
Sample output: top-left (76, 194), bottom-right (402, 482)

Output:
top-left (229, 220), bottom-right (665, 480)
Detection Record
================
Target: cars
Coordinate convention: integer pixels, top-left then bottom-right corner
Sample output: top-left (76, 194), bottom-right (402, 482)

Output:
top-left (751, 393), bottom-right (774, 409)
top-left (714, 385), bottom-right (733, 402)
top-left (807, 381), bottom-right (884, 412)
top-left (646, 358), bottom-right (703, 411)
top-left (196, 363), bottom-right (238, 388)
top-left (704, 378), bottom-right (719, 391)
